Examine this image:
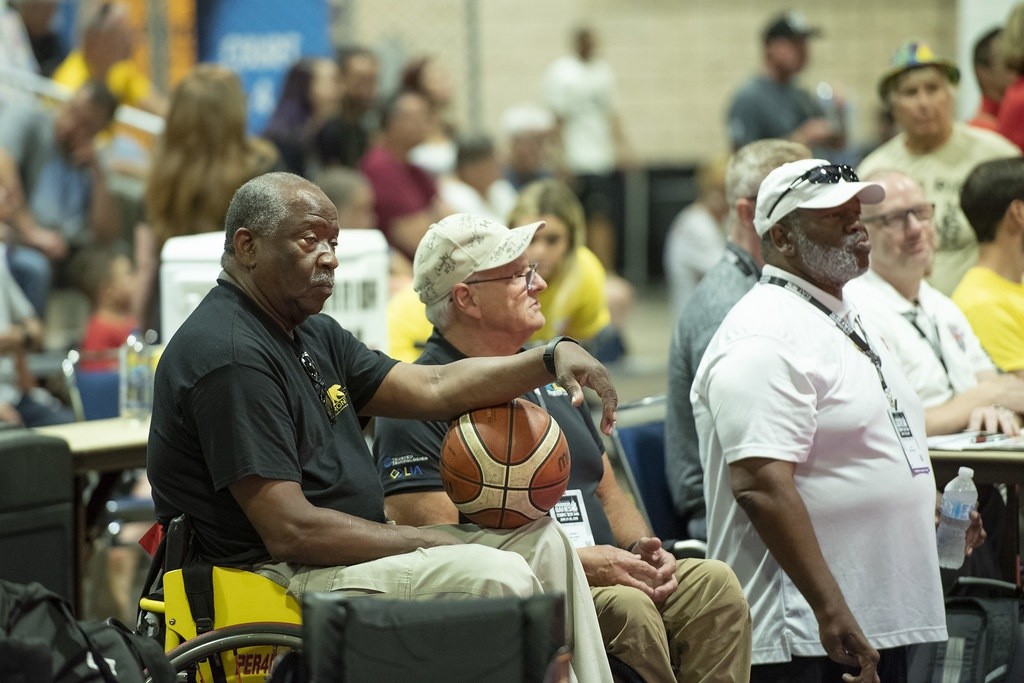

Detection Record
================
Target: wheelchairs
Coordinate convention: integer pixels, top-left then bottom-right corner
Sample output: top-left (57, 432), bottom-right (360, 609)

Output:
top-left (124, 506), bottom-right (301, 682)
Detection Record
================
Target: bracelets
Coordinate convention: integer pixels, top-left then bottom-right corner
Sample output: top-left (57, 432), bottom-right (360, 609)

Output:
top-left (542, 334), bottom-right (581, 376)
top-left (626, 540), bottom-right (638, 551)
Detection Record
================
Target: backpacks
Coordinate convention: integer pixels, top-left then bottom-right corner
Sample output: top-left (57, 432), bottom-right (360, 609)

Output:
top-left (903, 575), bottom-right (1024, 683)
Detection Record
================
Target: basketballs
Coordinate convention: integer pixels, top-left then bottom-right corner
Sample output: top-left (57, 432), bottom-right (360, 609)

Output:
top-left (440, 395), bottom-right (571, 532)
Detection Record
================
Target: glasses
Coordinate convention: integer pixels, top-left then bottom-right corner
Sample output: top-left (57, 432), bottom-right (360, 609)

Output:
top-left (766, 164), bottom-right (860, 221)
top-left (866, 201), bottom-right (936, 227)
top-left (449, 263), bottom-right (540, 304)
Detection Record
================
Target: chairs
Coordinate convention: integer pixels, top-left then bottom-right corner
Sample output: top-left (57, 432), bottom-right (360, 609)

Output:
top-left (613, 393), bottom-right (1024, 681)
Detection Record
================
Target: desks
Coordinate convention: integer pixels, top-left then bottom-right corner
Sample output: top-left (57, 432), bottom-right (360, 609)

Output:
top-left (27, 420), bottom-right (149, 533)
top-left (928, 435), bottom-right (1024, 593)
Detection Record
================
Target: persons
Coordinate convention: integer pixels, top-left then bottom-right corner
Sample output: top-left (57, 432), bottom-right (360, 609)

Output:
top-left (0, 0), bottom-right (1024, 683)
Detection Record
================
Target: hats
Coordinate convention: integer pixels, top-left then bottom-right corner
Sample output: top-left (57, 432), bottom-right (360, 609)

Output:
top-left (752, 158), bottom-right (887, 240)
top-left (876, 43), bottom-right (963, 109)
top-left (761, 10), bottom-right (824, 44)
top-left (412, 212), bottom-right (548, 307)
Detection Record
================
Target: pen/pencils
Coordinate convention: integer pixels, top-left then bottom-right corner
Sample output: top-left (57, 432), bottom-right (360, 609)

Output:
top-left (972, 433), bottom-right (1007, 444)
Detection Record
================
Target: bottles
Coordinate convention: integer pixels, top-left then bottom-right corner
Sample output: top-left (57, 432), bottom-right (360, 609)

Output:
top-left (117, 328), bottom-right (152, 422)
top-left (936, 466), bottom-right (978, 569)
